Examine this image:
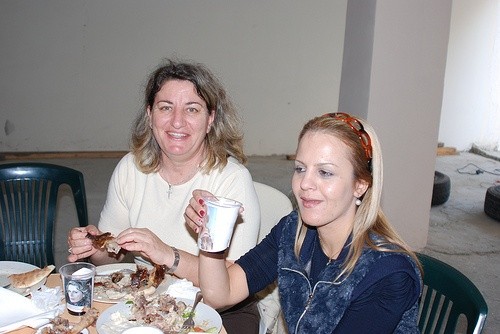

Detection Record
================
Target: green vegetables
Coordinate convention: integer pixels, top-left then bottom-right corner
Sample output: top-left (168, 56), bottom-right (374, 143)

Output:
top-left (126, 301), bottom-right (196, 318)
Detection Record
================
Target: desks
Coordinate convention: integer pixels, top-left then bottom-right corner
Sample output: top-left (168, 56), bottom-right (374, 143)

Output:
top-left (0, 272), bottom-right (227, 334)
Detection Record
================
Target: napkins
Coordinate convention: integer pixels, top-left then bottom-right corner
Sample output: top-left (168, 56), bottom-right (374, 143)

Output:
top-left (166, 278), bottom-right (203, 302)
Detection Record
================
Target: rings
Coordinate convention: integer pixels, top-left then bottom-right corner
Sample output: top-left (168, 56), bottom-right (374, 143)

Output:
top-left (68, 248), bottom-right (74, 255)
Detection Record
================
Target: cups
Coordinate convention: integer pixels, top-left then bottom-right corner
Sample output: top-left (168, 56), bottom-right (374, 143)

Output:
top-left (59, 262), bottom-right (96, 316)
top-left (196, 198), bottom-right (240, 252)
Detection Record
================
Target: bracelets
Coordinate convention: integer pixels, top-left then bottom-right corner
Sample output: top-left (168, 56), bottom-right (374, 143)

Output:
top-left (163, 246), bottom-right (180, 275)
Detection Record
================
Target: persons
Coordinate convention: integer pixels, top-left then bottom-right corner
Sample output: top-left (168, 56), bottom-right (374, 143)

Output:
top-left (184, 111), bottom-right (422, 334)
top-left (66, 60), bottom-right (261, 334)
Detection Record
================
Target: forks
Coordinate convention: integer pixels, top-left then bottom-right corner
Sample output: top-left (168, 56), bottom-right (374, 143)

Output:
top-left (180, 291), bottom-right (204, 330)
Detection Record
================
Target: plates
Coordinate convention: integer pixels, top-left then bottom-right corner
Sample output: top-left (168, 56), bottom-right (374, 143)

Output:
top-left (86, 263), bottom-right (171, 304)
top-left (121, 327), bottom-right (164, 334)
top-left (36, 321), bottom-right (89, 334)
top-left (0, 260), bottom-right (47, 298)
top-left (96, 298), bottom-right (222, 334)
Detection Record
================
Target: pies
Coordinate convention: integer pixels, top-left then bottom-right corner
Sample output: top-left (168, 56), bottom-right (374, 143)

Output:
top-left (7, 264), bottom-right (55, 288)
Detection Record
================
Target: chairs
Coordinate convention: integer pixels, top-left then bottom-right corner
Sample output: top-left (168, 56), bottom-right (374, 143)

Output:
top-left (251, 179), bottom-right (295, 334)
top-left (0, 162), bottom-right (91, 274)
top-left (410, 250), bottom-right (488, 334)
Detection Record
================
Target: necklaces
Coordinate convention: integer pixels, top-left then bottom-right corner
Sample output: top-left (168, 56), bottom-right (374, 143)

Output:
top-left (161, 163), bottom-right (196, 198)
